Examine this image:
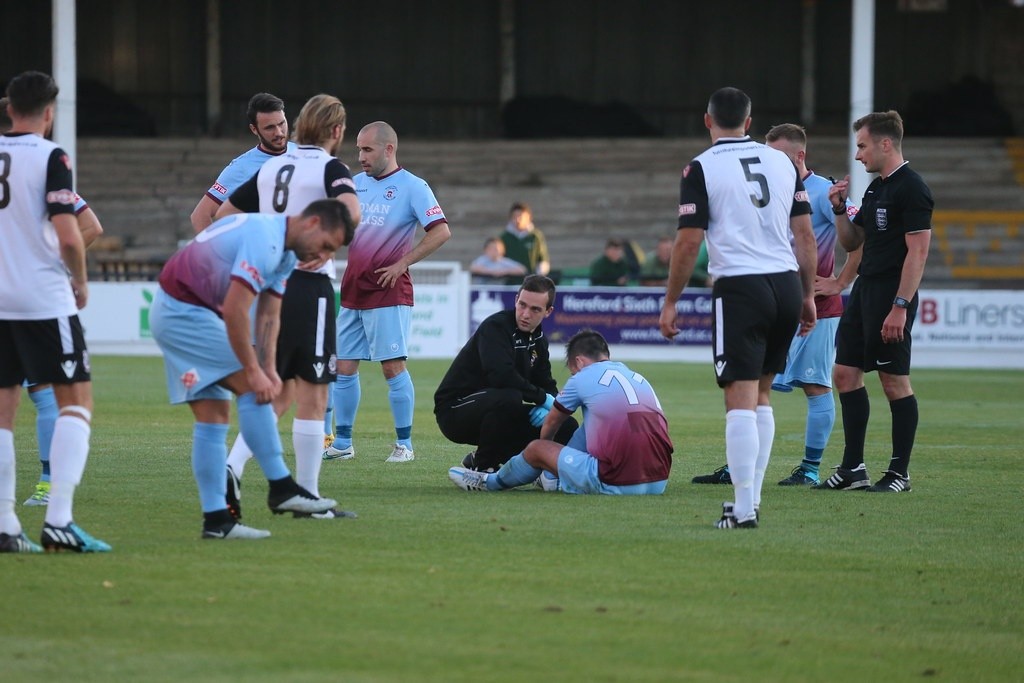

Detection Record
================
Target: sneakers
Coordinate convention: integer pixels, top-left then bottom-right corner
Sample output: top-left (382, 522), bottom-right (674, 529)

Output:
top-left (226, 464), bottom-right (242, 525)
top-left (40, 521), bottom-right (112, 554)
top-left (268, 487), bottom-right (338, 514)
top-left (460, 453), bottom-right (504, 474)
top-left (535, 471), bottom-right (558, 492)
top-left (322, 446), bottom-right (354, 460)
top-left (0, 532), bottom-right (45, 554)
top-left (778, 459), bottom-right (821, 486)
top-left (810, 462), bottom-right (871, 490)
top-left (722, 502), bottom-right (760, 522)
top-left (714, 516), bottom-right (757, 530)
top-left (448, 467), bottom-right (488, 491)
top-left (866, 470), bottom-right (913, 493)
top-left (293, 508), bottom-right (358, 519)
top-left (324, 434), bottom-right (334, 448)
top-left (22, 474), bottom-right (50, 506)
top-left (384, 443), bottom-right (414, 463)
top-left (201, 519), bottom-right (271, 540)
top-left (692, 464), bottom-right (732, 484)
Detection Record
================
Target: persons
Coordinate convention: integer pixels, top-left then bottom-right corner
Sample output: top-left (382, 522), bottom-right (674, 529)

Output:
top-left (214, 95), bottom-right (362, 521)
top-left (433, 275), bottom-right (556, 475)
top-left (640, 237), bottom-right (714, 290)
top-left (660, 87), bottom-right (818, 531)
top-left (146, 198), bottom-right (358, 540)
top-left (468, 236), bottom-right (526, 277)
top-left (588, 236), bottom-right (632, 287)
top-left (321, 120), bottom-right (451, 465)
top-left (691, 124), bottom-right (863, 488)
top-left (1, 71), bottom-right (112, 555)
top-left (191, 91), bottom-right (336, 449)
top-left (811, 110), bottom-right (932, 495)
top-left (447, 328), bottom-right (674, 495)
top-left (499, 199), bottom-right (552, 285)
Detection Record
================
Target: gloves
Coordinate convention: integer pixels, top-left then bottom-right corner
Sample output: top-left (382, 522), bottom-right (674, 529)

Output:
top-left (528, 393), bottom-right (555, 428)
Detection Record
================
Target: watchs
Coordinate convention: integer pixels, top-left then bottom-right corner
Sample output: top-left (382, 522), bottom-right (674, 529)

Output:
top-left (891, 295), bottom-right (911, 309)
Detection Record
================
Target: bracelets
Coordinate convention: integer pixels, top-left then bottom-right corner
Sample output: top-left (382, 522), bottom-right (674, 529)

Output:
top-left (831, 204), bottom-right (847, 217)
